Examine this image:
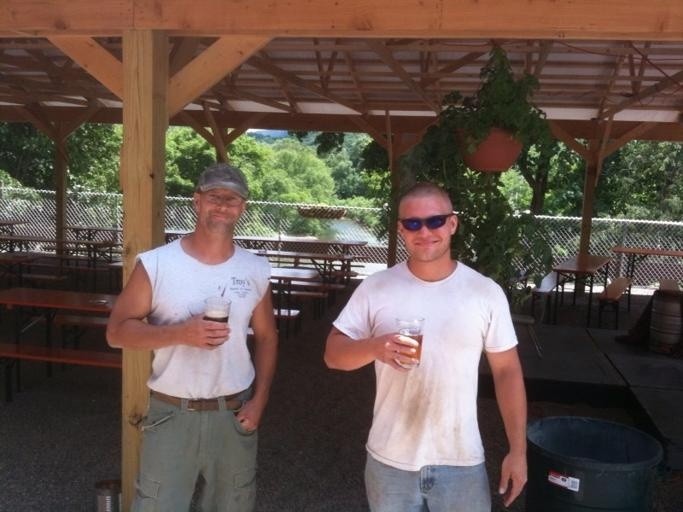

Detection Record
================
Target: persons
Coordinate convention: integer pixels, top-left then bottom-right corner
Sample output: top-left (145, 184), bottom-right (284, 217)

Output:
top-left (321, 183), bottom-right (531, 512)
top-left (104, 159), bottom-right (281, 511)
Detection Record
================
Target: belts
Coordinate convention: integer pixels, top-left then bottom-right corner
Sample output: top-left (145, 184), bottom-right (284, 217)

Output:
top-left (149, 383), bottom-right (253, 411)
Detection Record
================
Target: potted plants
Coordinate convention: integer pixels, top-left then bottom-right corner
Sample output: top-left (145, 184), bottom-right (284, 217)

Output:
top-left (378, 43), bottom-right (552, 306)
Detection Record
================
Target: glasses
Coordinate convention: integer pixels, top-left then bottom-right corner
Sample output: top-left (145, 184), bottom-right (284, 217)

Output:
top-left (396, 213), bottom-right (455, 232)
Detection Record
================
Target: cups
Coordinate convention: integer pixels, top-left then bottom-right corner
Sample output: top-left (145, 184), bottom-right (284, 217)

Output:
top-left (394, 314), bottom-right (425, 368)
top-left (200, 296), bottom-right (229, 320)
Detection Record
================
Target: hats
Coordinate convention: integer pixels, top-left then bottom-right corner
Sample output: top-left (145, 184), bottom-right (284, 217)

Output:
top-left (195, 163), bottom-right (252, 201)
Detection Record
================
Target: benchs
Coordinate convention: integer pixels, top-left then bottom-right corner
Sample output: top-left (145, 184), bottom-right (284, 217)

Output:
top-left (0, 220), bottom-right (369, 402)
top-left (598, 278), bottom-right (634, 328)
top-left (531, 272), bottom-right (566, 324)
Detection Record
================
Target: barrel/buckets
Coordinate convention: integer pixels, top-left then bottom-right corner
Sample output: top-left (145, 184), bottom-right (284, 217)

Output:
top-left (649, 290), bottom-right (683, 354)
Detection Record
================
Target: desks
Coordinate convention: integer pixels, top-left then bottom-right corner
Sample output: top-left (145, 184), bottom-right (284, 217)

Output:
top-left (613, 247), bottom-right (683, 295)
top-left (552, 255), bottom-right (612, 328)
